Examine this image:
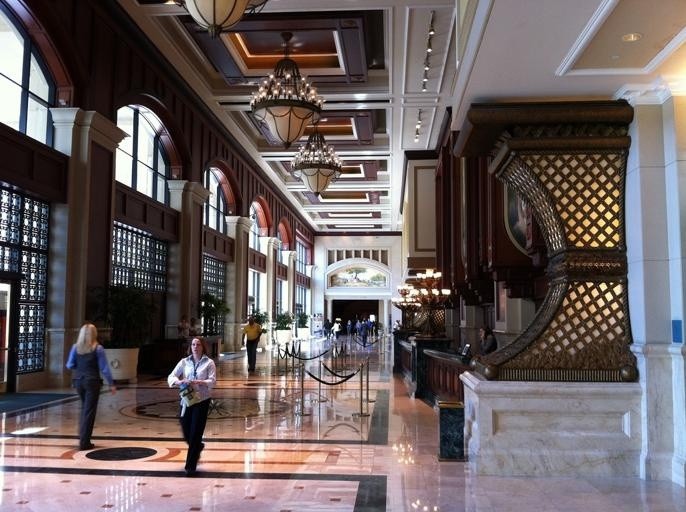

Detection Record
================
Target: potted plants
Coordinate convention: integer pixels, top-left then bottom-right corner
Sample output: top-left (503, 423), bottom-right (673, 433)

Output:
top-left (253, 308), bottom-right (270, 348)
top-left (94, 282), bottom-right (153, 385)
top-left (297, 313), bottom-right (309, 341)
top-left (275, 313), bottom-right (293, 344)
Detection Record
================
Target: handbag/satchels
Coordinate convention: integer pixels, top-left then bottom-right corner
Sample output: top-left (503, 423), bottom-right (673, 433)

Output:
top-left (178, 386), bottom-right (200, 407)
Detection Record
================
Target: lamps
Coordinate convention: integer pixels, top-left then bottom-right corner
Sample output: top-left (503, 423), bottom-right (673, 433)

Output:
top-left (163, 0), bottom-right (267, 42)
top-left (422, 25), bottom-right (435, 92)
top-left (291, 115), bottom-right (343, 197)
top-left (411, 269), bottom-right (451, 337)
top-left (415, 119), bottom-right (421, 143)
top-left (250, 32), bottom-right (323, 149)
top-left (392, 285), bottom-right (421, 336)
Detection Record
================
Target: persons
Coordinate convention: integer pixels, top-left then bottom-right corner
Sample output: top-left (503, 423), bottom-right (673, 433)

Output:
top-left (166, 336), bottom-right (217, 477)
top-left (323, 316), bottom-right (380, 347)
top-left (65, 322), bottom-right (116, 451)
top-left (471, 325), bottom-right (497, 357)
top-left (241, 316), bottom-right (262, 372)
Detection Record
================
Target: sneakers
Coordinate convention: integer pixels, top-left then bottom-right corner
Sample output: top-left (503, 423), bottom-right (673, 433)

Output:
top-left (185, 468), bottom-right (195, 476)
top-left (81, 444), bottom-right (94, 450)
top-left (200, 443), bottom-right (204, 451)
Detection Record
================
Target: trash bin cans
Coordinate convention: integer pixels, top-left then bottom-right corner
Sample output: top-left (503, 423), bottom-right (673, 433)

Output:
top-left (437, 401), bottom-right (465, 462)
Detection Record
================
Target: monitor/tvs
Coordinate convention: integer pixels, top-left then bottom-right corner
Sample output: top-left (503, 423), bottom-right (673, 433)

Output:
top-left (462, 343), bottom-right (471, 356)
